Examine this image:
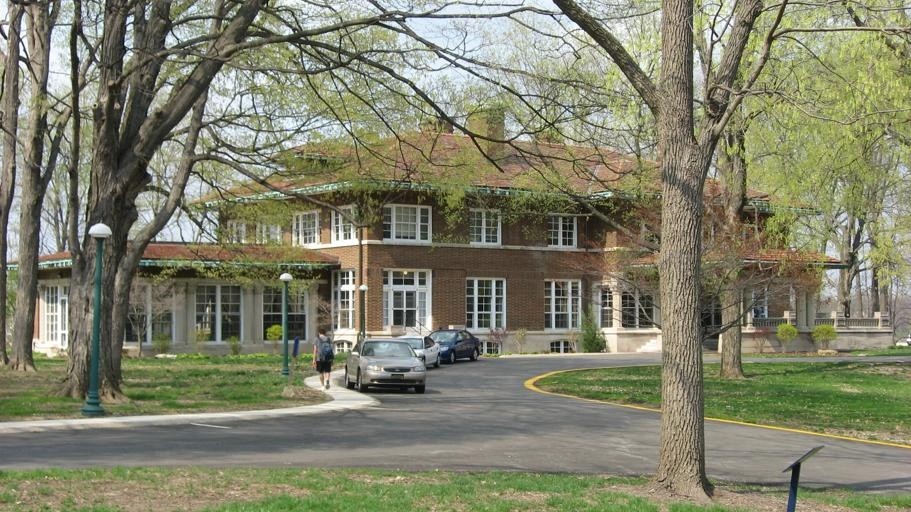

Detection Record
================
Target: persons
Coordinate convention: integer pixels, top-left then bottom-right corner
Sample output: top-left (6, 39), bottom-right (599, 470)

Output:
top-left (313, 329), bottom-right (331, 389)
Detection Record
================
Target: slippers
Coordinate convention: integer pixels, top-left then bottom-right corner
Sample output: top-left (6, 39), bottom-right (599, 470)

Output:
top-left (316, 335), bottom-right (333, 363)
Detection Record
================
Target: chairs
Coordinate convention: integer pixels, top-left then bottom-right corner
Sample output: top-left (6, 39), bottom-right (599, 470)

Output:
top-left (325, 379), bottom-right (330, 389)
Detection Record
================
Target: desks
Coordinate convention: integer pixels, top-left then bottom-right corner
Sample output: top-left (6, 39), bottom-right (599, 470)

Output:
top-left (359, 284), bottom-right (368, 339)
top-left (280, 273), bottom-right (293, 375)
top-left (82, 223), bottom-right (112, 415)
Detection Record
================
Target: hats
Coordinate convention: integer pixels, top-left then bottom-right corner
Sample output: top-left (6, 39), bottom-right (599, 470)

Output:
top-left (345, 329), bottom-right (481, 393)
